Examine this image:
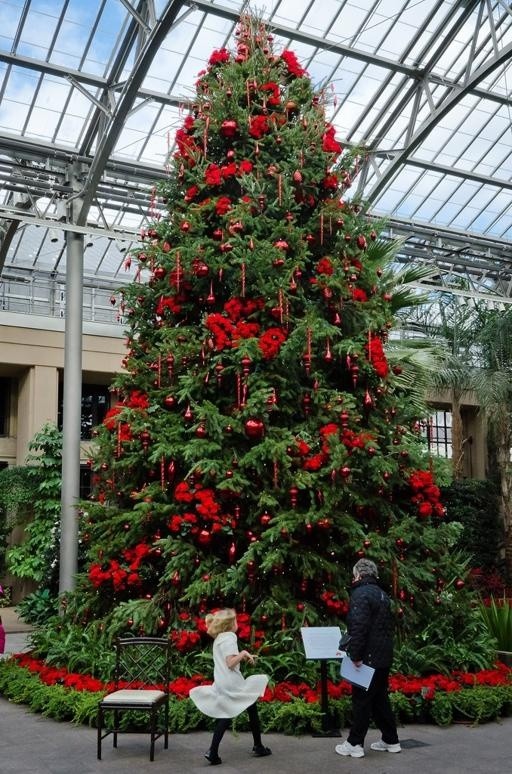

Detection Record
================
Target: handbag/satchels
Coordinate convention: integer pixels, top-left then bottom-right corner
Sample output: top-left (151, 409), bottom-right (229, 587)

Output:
top-left (338, 632), bottom-right (380, 669)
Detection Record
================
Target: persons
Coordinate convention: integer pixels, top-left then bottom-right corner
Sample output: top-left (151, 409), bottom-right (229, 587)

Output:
top-left (332, 556), bottom-right (406, 758)
top-left (188, 608), bottom-right (274, 764)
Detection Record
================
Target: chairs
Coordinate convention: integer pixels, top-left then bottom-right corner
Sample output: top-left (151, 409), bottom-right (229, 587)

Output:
top-left (97, 636), bottom-right (172, 762)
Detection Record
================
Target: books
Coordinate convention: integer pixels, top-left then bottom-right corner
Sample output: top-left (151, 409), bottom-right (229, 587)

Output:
top-left (338, 656), bottom-right (376, 688)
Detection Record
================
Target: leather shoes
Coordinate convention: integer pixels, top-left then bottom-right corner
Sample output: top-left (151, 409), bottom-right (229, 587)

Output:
top-left (203, 748), bottom-right (222, 766)
top-left (251, 745), bottom-right (273, 757)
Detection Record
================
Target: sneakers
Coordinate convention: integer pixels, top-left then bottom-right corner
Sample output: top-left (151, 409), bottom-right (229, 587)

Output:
top-left (369, 740), bottom-right (403, 753)
top-left (335, 739), bottom-right (365, 758)
